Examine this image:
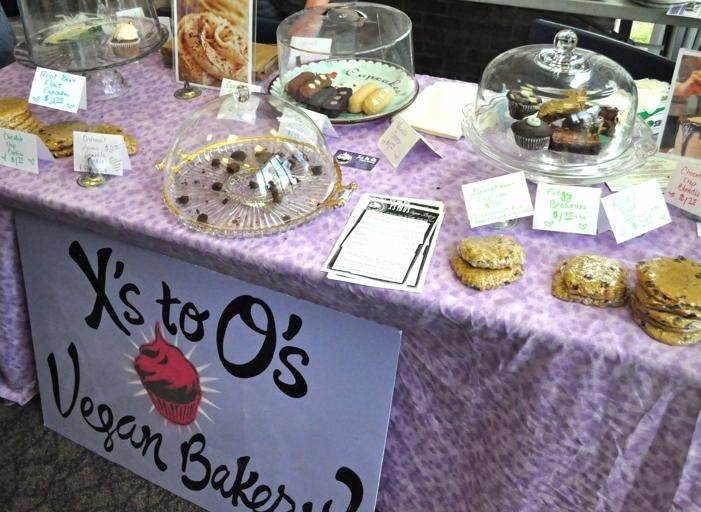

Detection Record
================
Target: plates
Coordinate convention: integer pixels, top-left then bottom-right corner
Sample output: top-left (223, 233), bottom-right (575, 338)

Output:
top-left (266, 56), bottom-right (418, 125)
top-left (460, 91), bottom-right (658, 187)
top-left (163, 135), bottom-right (342, 240)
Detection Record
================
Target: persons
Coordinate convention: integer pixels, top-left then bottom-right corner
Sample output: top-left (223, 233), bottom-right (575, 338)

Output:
top-left (264, 0), bottom-right (358, 58)
top-left (671, 68), bottom-right (701, 99)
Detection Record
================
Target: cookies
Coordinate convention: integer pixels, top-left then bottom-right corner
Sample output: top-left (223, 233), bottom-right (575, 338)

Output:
top-left (0, 98), bottom-right (138, 157)
top-left (450, 235), bottom-right (701, 346)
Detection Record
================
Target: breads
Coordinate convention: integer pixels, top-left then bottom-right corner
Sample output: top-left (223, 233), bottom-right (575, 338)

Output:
top-left (506, 86), bottom-right (620, 154)
top-left (286, 72), bottom-right (391, 115)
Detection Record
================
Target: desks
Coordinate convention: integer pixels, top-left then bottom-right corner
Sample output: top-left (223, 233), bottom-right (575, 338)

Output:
top-left (0, 31), bottom-right (699, 509)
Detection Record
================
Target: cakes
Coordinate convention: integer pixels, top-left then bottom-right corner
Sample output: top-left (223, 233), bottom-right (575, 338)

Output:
top-left (108, 23), bottom-right (140, 59)
top-left (132, 321), bottom-right (202, 426)
